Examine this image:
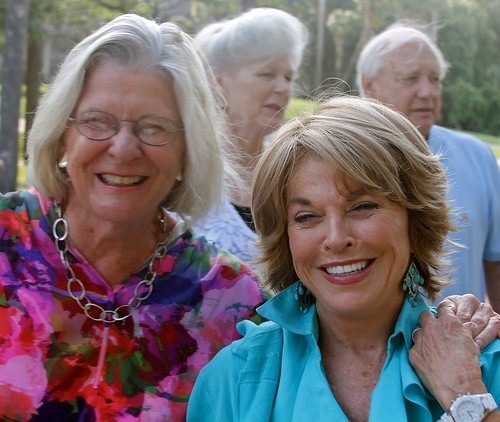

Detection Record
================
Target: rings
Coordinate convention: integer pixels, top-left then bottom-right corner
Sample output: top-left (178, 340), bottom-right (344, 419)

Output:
top-left (411, 328), bottom-right (423, 342)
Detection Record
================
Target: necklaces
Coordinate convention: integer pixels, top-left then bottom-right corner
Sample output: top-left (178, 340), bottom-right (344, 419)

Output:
top-left (53, 195), bottom-right (169, 323)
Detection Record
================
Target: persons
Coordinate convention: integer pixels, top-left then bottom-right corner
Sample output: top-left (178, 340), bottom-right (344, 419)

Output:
top-left (163, 7), bottom-right (311, 296)
top-left (186, 93), bottom-right (500, 422)
top-left (359, 19), bottom-right (500, 307)
top-left (1, 13), bottom-right (500, 422)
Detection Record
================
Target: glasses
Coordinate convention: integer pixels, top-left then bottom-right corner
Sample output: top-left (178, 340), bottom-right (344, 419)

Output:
top-left (65, 109), bottom-right (184, 146)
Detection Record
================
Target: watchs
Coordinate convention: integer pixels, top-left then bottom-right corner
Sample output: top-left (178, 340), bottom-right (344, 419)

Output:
top-left (435, 392), bottom-right (498, 422)
